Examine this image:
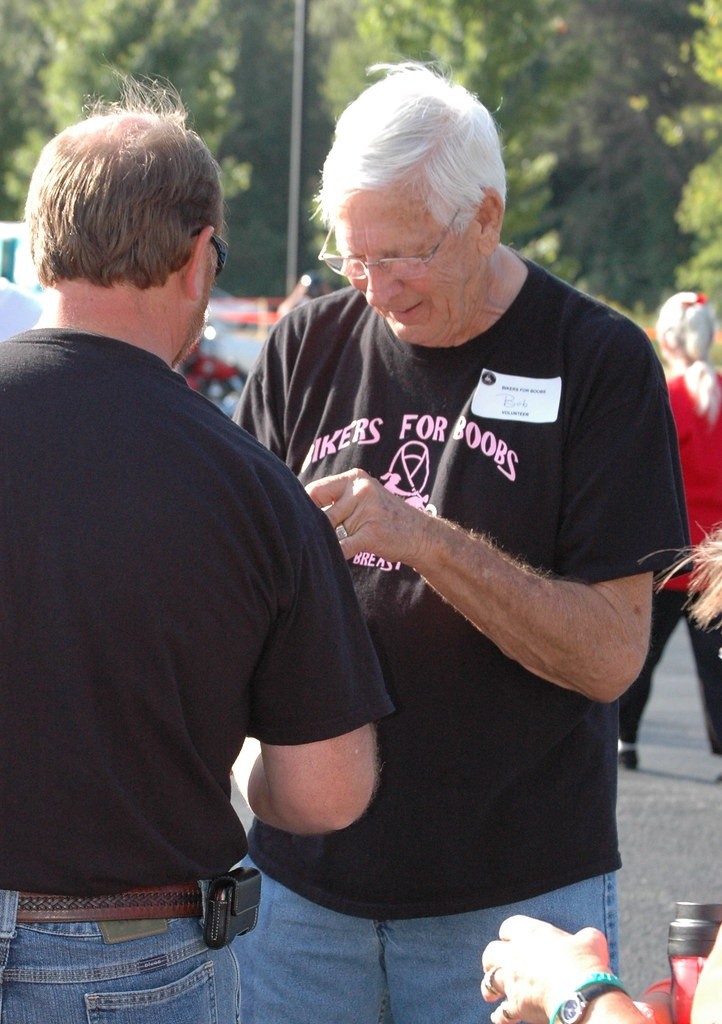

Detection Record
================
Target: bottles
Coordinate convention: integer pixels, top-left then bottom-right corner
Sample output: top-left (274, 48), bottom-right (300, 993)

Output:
top-left (667, 902), bottom-right (722, 1024)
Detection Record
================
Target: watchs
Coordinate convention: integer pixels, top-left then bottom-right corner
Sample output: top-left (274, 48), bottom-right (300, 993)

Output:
top-left (550, 980), bottom-right (631, 1024)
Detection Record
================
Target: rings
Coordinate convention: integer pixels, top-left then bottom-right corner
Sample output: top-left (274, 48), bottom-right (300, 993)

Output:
top-left (484, 966), bottom-right (504, 995)
top-left (335, 523), bottom-right (348, 540)
top-left (501, 1000), bottom-right (521, 1019)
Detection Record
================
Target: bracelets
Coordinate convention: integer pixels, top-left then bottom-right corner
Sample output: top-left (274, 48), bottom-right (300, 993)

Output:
top-left (549, 972), bottom-right (629, 1024)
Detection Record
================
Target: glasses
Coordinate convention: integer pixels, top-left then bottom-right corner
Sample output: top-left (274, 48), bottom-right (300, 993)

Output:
top-left (188, 228), bottom-right (230, 275)
top-left (317, 206), bottom-right (460, 279)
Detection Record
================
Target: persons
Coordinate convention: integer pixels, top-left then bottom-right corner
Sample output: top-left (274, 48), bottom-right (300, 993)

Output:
top-left (618, 289), bottom-right (722, 783)
top-left (0, 71), bottom-right (396, 1024)
top-left (229, 61), bottom-right (694, 1024)
top-left (480, 914), bottom-right (722, 1024)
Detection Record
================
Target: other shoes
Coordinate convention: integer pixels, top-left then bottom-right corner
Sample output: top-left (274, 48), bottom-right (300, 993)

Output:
top-left (618, 750), bottom-right (637, 769)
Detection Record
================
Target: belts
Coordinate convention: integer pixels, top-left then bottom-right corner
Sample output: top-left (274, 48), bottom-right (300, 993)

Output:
top-left (15, 881), bottom-right (205, 922)
